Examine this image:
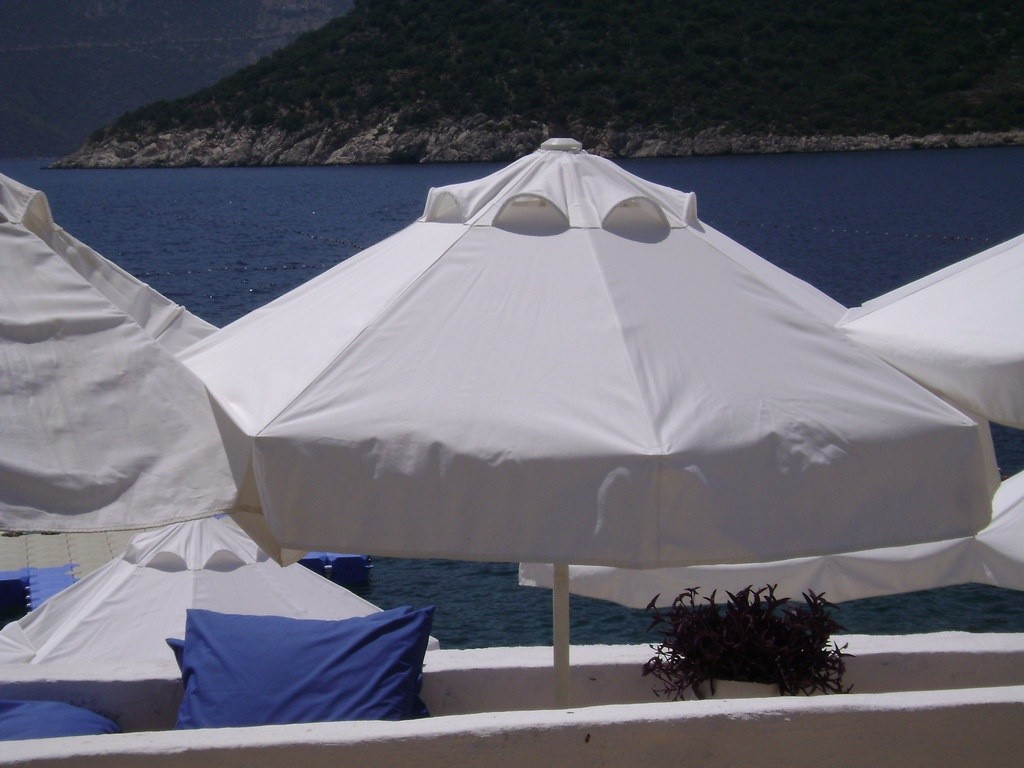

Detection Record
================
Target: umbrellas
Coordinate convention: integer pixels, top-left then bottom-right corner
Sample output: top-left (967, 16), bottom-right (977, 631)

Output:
top-left (515, 459), bottom-right (1023, 612)
top-left (173, 129), bottom-right (997, 707)
top-left (1, 173), bottom-right (242, 537)
top-left (829, 228), bottom-right (1023, 432)
top-left (2, 515), bottom-right (440, 678)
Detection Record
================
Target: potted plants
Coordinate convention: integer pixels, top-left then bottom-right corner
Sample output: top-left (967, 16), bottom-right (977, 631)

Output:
top-left (642, 584), bottom-right (856, 702)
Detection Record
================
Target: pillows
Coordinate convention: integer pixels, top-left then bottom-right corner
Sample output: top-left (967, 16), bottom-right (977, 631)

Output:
top-left (0, 698), bottom-right (123, 742)
top-left (167, 605), bottom-right (439, 729)
